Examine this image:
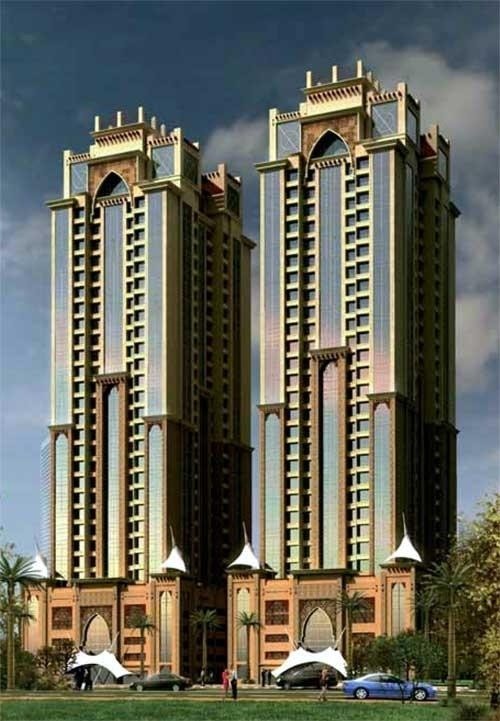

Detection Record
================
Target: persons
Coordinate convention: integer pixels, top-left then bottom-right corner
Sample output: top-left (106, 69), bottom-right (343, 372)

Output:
top-left (317, 670), bottom-right (330, 703)
top-left (261, 668), bottom-right (267, 688)
top-left (229, 665), bottom-right (238, 700)
top-left (268, 670), bottom-right (273, 687)
top-left (222, 670), bottom-right (231, 699)
